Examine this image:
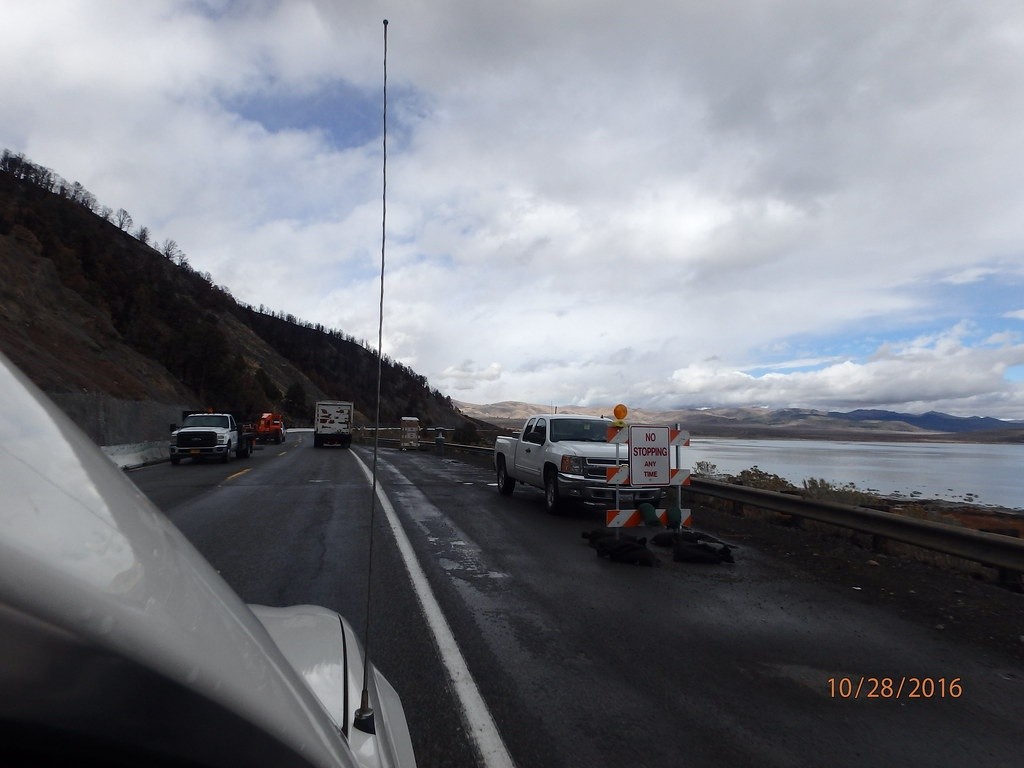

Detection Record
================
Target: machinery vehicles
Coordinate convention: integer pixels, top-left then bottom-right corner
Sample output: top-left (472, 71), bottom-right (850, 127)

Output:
top-left (245, 412), bottom-right (287, 443)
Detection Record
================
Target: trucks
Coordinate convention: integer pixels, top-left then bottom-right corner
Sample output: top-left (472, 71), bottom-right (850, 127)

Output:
top-left (313, 400), bottom-right (357, 450)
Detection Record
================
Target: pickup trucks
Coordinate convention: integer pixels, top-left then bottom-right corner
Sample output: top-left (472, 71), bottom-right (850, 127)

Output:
top-left (493, 411), bottom-right (649, 516)
top-left (167, 409), bottom-right (256, 463)
